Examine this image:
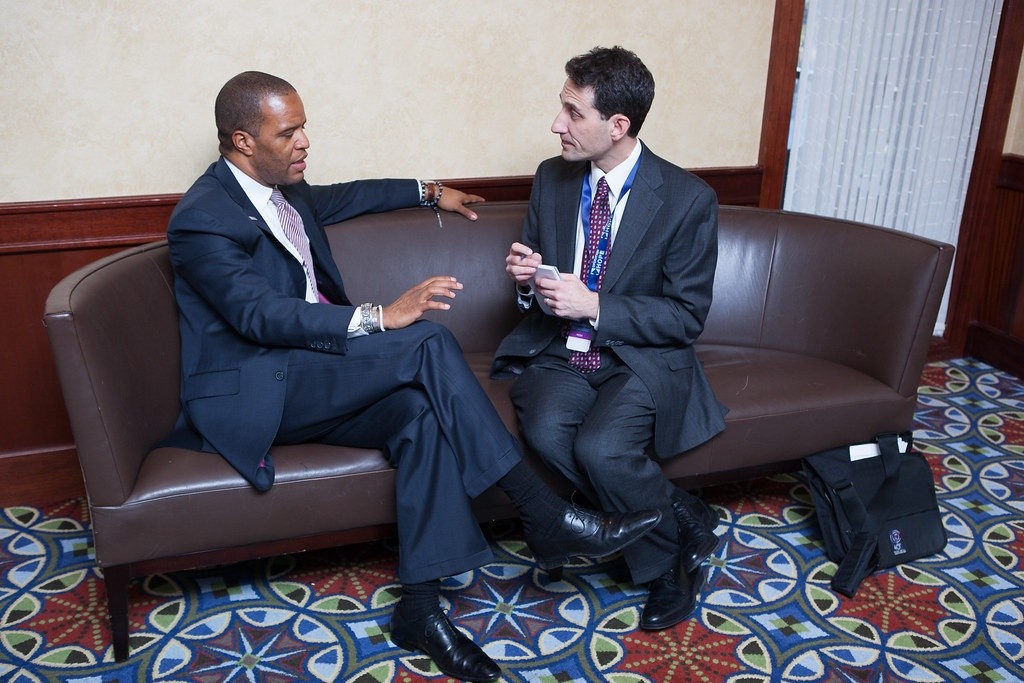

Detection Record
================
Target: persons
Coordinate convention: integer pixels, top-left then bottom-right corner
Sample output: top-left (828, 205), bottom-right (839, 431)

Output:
top-left (167, 71), bottom-right (662, 683)
top-left (488, 46), bottom-right (731, 630)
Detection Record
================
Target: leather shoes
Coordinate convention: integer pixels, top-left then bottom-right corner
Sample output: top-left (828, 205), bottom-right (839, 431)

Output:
top-left (391, 602), bottom-right (502, 683)
top-left (525, 490), bottom-right (662, 570)
top-left (640, 563), bottom-right (703, 630)
top-left (672, 496), bottom-right (721, 573)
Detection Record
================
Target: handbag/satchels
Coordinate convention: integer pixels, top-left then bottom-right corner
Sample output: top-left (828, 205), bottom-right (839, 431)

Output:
top-left (802, 427), bottom-right (948, 599)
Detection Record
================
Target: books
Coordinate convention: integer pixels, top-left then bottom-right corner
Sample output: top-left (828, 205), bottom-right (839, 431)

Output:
top-left (527, 266), bottom-right (579, 321)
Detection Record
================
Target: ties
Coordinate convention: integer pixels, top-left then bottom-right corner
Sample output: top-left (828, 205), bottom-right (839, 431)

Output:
top-left (269, 188), bottom-right (319, 304)
top-left (567, 175), bottom-right (612, 375)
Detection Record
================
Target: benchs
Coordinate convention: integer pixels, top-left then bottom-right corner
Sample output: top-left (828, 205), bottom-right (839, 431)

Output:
top-left (44, 203), bottom-right (954, 663)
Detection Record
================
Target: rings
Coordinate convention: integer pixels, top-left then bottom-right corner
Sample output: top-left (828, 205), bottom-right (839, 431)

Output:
top-left (546, 298), bottom-right (549, 305)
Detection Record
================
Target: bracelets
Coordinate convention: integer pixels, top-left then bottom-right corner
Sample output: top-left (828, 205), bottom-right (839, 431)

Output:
top-left (421, 181), bottom-right (443, 227)
top-left (360, 302), bottom-right (386, 334)
